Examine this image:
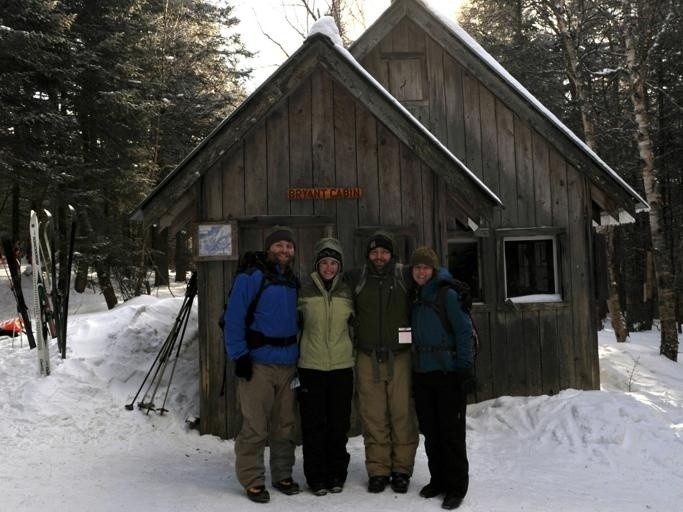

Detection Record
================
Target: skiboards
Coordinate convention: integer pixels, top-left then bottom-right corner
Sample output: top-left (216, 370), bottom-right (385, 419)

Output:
top-left (4, 204), bottom-right (76, 376)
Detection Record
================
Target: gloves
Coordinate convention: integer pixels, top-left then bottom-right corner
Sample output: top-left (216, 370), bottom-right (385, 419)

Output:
top-left (232, 352), bottom-right (256, 381)
top-left (460, 373), bottom-right (481, 395)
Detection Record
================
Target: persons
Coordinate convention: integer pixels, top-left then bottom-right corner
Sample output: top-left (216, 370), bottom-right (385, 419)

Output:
top-left (296, 236), bottom-right (356, 496)
top-left (407, 246), bottom-right (477, 510)
top-left (223, 225), bottom-right (302, 503)
top-left (342, 231), bottom-right (420, 493)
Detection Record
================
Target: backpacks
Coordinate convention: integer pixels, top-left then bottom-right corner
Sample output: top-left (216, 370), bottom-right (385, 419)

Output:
top-left (217, 250), bottom-right (273, 334)
top-left (435, 279), bottom-right (481, 360)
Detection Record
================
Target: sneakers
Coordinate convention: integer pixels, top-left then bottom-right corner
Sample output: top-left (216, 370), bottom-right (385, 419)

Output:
top-left (388, 475), bottom-right (411, 495)
top-left (310, 484), bottom-right (343, 498)
top-left (363, 473), bottom-right (390, 493)
top-left (246, 483), bottom-right (270, 502)
top-left (418, 482), bottom-right (442, 500)
top-left (272, 477), bottom-right (300, 496)
top-left (442, 491), bottom-right (464, 510)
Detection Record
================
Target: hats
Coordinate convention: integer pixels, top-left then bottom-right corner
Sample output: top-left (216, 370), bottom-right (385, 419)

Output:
top-left (263, 224), bottom-right (297, 252)
top-left (312, 237), bottom-right (344, 267)
top-left (364, 230), bottom-right (398, 256)
top-left (409, 246), bottom-right (440, 270)
